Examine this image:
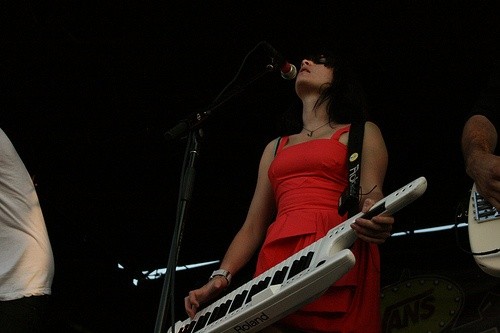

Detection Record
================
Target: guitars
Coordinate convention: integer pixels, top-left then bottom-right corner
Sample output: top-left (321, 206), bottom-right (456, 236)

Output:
top-left (467, 172), bottom-right (500, 274)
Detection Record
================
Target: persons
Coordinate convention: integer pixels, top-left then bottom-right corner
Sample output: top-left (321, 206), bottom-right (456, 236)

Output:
top-left (185, 46), bottom-right (394, 333)
top-left (461, 58), bottom-right (500, 213)
top-left (0, 128), bottom-right (54, 333)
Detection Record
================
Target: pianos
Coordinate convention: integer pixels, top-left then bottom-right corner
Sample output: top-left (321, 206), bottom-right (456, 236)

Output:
top-left (167, 176), bottom-right (428, 333)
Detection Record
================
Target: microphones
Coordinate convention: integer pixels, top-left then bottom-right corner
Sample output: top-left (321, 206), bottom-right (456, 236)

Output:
top-left (264, 41), bottom-right (297, 80)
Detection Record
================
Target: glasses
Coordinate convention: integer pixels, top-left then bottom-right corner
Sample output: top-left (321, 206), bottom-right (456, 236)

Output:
top-left (295, 50), bottom-right (337, 67)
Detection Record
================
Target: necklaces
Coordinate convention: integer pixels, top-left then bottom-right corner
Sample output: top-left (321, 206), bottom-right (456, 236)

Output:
top-left (303, 121), bottom-right (331, 137)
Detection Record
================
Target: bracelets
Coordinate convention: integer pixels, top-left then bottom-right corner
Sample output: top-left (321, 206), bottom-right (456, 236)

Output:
top-left (210, 270), bottom-right (233, 287)
